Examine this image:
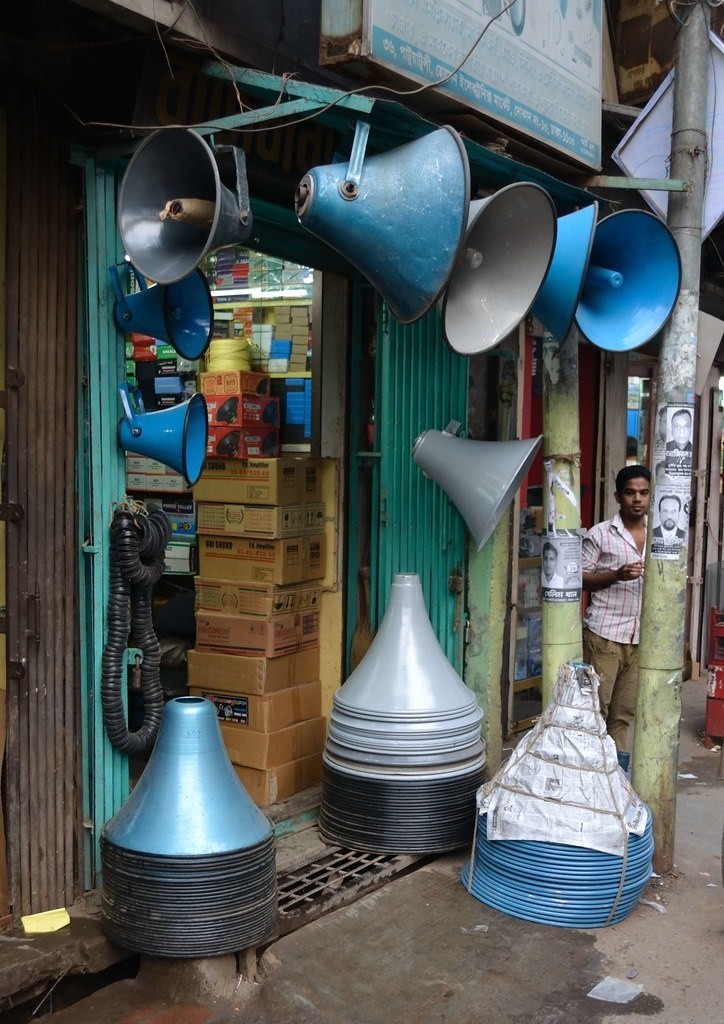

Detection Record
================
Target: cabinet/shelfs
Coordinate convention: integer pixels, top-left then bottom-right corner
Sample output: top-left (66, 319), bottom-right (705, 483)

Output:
top-left (195, 299), bottom-right (313, 454)
top-left (512, 527), bottom-right (543, 735)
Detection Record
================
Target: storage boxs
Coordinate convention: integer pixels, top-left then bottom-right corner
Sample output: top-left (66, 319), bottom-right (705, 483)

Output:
top-left (121, 242), bottom-right (328, 804)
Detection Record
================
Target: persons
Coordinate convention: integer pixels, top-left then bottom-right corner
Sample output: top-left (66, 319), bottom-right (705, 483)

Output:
top-left (542, 544), bottom-right (559, 589)
top-left (623, 434), bottom-right (639, 465)
top-left (580, 466), bottom-right (651, 774)
top-left (650, 399), bottom-right (693, 539)
